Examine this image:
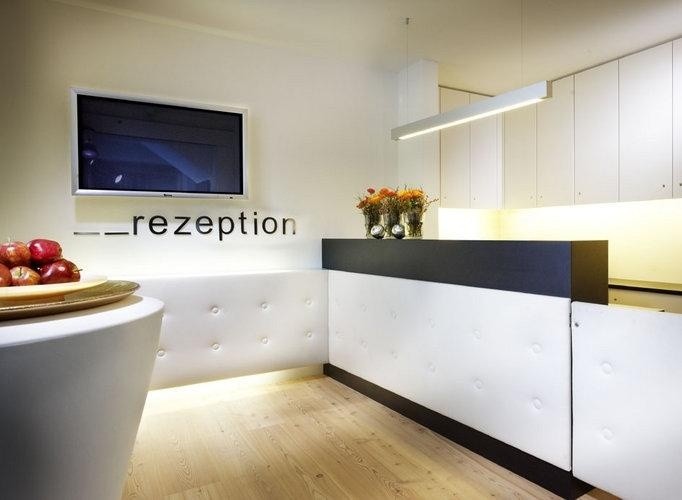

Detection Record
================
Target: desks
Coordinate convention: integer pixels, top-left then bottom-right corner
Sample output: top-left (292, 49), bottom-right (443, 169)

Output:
top-left (0, 294), bottom-right (165, 500)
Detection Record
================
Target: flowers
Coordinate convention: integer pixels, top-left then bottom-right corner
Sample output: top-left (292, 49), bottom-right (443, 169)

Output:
top-left (356, 185), bottom-right (382, 225)
top-left (394, 189), bottom-right (438, 213)
top-left (371, 186), bottom-right (405, 214)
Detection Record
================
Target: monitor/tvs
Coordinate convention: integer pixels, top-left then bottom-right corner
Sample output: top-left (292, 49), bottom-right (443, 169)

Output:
top-left (69, 85), bottom-right (251, 201)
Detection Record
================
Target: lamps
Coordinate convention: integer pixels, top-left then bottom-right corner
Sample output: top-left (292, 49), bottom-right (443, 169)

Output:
top-left (391, 81), bottom-right (552, 140)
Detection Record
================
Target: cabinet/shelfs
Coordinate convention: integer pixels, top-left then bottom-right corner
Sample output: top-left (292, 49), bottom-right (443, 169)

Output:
top-left (575, 59), bottom-right (620, 205)
top-left (619, 38), bottom-right (682, 201)
top-left (439, 86), bottom-right (503, 210)
top-left (503, 74), bottom-right (575, 210)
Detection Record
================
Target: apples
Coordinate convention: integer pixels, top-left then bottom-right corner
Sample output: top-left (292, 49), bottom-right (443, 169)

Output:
top-left (0, 239), bottom-right (81, 287)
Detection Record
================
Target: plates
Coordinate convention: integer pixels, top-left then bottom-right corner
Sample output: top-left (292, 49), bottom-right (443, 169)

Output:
top-left (2, 280), bottom-right (110, 299)
top-left (1, 279), bottom-right (144, 318)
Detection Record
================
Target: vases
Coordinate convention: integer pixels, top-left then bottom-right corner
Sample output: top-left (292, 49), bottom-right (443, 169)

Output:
top-left (403, 212), bottom-right (424, 236)
top-left (364, 214), bottom-right (380, 238)
top-left (382, 212), bottom-right (400, 237)
